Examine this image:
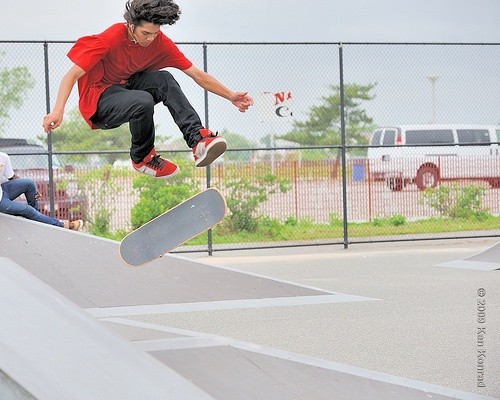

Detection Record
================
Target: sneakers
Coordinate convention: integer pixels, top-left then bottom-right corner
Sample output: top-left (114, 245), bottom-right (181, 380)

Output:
top-left (66, 220), bottom-right (83, 231)
top-left (192, 129), bottom-right (228, 167)
top-left (132, 148), bottom-right (180, 180)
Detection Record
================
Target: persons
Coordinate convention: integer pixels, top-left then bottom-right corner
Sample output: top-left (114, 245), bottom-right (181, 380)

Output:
top-left (43, 0), bottom-right (254, 179)
top-left (0, 152), bottom-right (84, 231)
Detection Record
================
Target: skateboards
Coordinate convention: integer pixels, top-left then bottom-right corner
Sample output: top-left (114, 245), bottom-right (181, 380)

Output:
top-left (120, 188), bottom-right (227, 266)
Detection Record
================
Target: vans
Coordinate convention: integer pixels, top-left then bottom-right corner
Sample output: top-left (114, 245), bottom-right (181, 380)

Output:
top-left (366, 125), bottom-right (500, 191)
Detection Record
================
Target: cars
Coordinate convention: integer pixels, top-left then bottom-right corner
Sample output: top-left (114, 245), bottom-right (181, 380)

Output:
top-left (2, 145), bottom-right (88, 221)
